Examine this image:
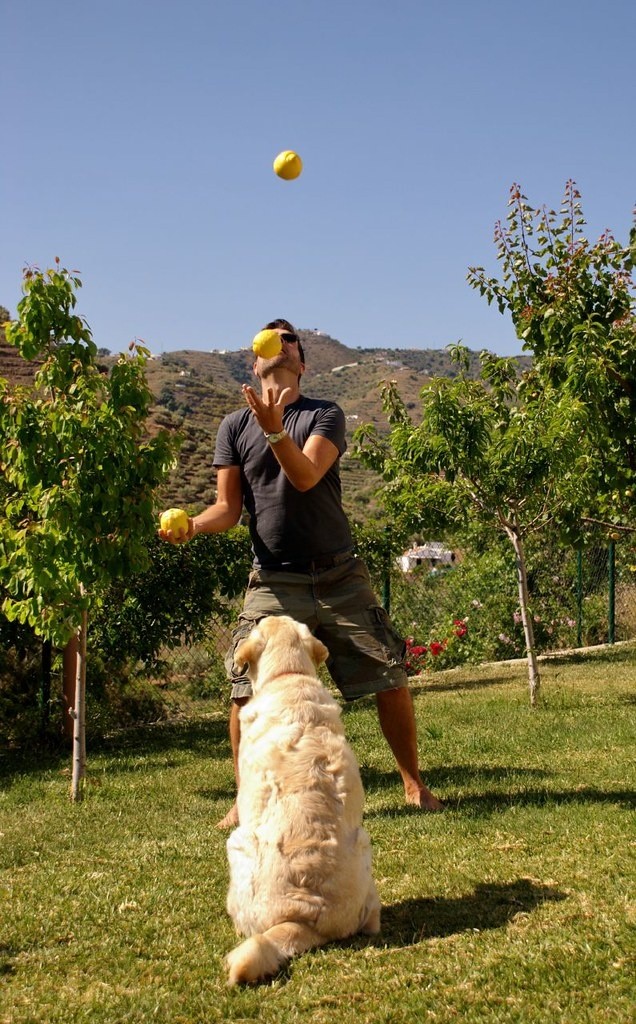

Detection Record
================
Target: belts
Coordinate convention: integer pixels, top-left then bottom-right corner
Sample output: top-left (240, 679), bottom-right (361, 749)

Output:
top-left (254, 548), bottom-right (353, 575)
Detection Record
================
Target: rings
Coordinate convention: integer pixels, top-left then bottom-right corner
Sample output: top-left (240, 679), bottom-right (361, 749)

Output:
top-left (251, 403), bottom-right (256, 406)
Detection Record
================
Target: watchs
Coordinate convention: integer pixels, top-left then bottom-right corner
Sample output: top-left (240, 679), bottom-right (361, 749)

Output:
top-left (263, 429), bottom-right (288, 444)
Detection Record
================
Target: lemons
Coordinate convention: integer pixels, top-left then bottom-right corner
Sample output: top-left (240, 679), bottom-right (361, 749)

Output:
top-left (160, 508), bottom-right (190, 538)
top-left (273, 150), bottom-right (302, 179)
top-left (252, 330), bottom-right (282, 358)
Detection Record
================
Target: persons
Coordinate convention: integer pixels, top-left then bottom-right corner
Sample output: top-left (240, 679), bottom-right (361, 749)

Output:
top-left (155, 317), bottom-right (442, 831)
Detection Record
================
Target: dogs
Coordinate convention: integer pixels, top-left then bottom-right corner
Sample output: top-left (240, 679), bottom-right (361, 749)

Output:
top-left (223, 615), bottom-right (382, 992)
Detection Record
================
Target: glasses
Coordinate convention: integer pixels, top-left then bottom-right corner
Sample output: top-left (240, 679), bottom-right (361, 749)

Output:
top-left (281, 334), bottom-right (301, 345)
